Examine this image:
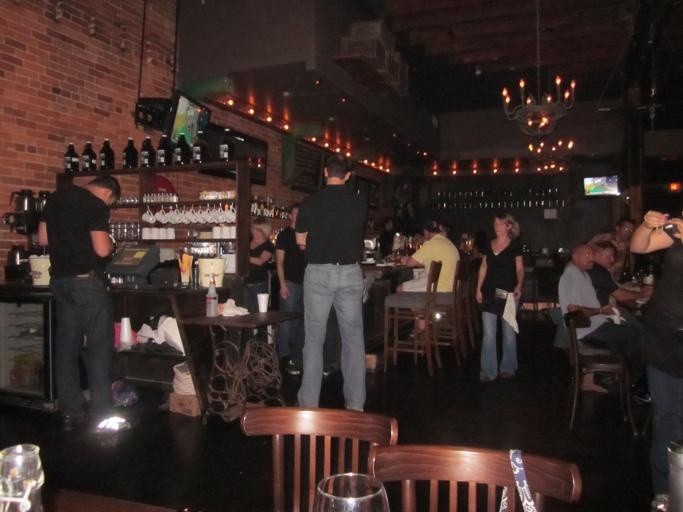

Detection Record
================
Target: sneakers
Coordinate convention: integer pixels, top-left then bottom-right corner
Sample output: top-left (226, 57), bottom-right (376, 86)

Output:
top-left (285, 358), bottom-right (302, 376)
top-left (323, 363), bottom-right (336, 376)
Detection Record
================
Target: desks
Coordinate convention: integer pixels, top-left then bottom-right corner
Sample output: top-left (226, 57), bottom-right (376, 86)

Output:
top-left (181, 310), bottom-right (297, 425)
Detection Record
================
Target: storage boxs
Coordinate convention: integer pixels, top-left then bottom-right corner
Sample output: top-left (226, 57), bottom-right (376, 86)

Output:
top-left (168, 391), bottom-right (200, 420)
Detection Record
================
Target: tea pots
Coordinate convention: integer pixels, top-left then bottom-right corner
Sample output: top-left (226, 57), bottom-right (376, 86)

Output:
top-left (35, 191), bottom-right (48, 211)
top-left (9, 188), bottom-right (34, 212)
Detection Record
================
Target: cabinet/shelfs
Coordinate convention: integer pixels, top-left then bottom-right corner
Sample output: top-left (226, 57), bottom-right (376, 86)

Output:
top-left (59, 174), bottom-right (142, 243)
top-left (142, 161), bottom-right (244, 293)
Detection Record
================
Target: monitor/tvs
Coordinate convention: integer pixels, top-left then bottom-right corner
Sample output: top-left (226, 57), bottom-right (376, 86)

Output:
top-left (159, 89), bottom-right (211, 149)
top-left (582, 173), bottom-right (623, 198)
top-left (109, 245), bottom-right (158, 274)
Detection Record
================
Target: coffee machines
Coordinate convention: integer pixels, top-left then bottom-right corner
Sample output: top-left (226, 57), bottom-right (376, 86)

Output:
top-left (0, 212), bottom-right (48, 280)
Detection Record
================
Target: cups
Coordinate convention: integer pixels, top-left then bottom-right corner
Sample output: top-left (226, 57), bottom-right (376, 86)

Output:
top-left (118, 316), bottom-right (131, 344)
top-left (197, 257), bottom-right (225, 288)
top-left (188, 267), bottom-right (198, 289)
top-left (27, 255), bottom-right (51, 285)
top-left (666, 438), bottom-right (682, 512)
top-left (256, 292), bottom-right (269, 314)
top-left (107, 189), bottom-right (237, 240)
top-left (310, 471), bottom-right (390, 511)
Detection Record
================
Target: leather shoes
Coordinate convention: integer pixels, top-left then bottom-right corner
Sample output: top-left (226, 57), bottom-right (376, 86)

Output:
top-left (479, 372), bottom-right (514, 384)
top-left (61, 411), bottom-right (88, 432)
top-left (581, 382), bottom-right (609, 394)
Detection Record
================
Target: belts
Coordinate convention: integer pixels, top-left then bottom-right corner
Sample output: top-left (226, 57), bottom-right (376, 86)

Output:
top-left (48, 265), bottom-right (96, 277)
top-left (311, 257), bottom-right (356, 266)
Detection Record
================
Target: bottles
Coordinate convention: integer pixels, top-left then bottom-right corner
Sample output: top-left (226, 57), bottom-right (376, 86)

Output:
top-left (0, 444), bottom-right (44, 512)
top-left (206, 275), bottom-right (217, 318)
top-left (249, 194), bottom-right (290, 219)
top-left (434, 187), bottom-right (562, 208)
top-left (64, 128), bottom-right (233, 171)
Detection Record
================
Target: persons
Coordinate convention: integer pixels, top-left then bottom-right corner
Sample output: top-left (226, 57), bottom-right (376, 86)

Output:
top-left (37, 175), bottom-right (140, 434)
top-left (556, 209), bottom-right (683, 512)
top-left (297, 155), bottom-right (368, 412)
top-left (378, 212), bottom-right (527, 384)
top-left (173, 103), bottom-right (197, 146)
top-left (276, 201), bottom-right (304, 376)
top-left (241, 216), bottom-right (275, 312)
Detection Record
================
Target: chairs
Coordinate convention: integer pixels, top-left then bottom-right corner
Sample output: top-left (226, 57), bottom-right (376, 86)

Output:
top-left (562, 310), bottom-right (623, 425)
top-left (363, 241), bottom-right (643, 379)
top-left (365, 443), bottom-right (585, 512)
top-left (237, 405), bottom-right (398, 510)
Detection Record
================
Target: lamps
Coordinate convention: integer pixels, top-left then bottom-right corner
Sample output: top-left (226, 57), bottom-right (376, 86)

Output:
top-left (497, 0), bottom-right (577, 138)
